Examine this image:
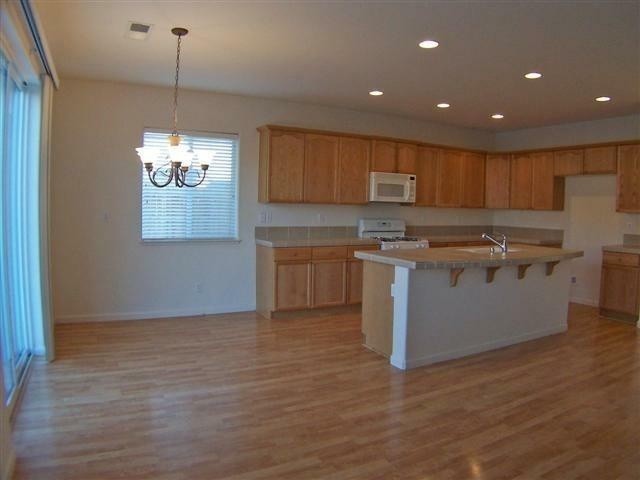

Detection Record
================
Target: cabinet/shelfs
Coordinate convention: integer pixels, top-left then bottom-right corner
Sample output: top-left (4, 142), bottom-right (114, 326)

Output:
top-left (257, 124), bottom-right (370, 204)
top-left (255, 244), bottom-right (381, 321)
top-left (615, 140), bottom-right (640, 214)
top-left (599, 249), bottom-right (640, 326)
top-left (370, 135), bottom-right (419, 174)
top-left (553, 141), bottom-right (617, 177)
top-left (401, 140), bottom-right (563, 210)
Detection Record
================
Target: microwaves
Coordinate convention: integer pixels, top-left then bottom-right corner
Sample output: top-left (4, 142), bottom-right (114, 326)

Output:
top-left (369, 171), bottom-right (417, 204)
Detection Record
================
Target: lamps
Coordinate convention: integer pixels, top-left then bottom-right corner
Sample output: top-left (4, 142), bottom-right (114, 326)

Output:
top-left (134, 27), bottom-right (216, 188)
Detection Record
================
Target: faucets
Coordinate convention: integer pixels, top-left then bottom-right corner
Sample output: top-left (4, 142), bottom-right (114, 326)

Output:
top-left (482, 232), bottom-right (507, 252)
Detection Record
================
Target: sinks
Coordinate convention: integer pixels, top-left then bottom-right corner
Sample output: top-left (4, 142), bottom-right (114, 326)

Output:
top-left (458, 247), bottom-right (523, 253)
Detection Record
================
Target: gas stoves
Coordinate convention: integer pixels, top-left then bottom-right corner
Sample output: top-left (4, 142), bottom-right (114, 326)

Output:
top-left (367, 236), bottom-right (429, 245)
